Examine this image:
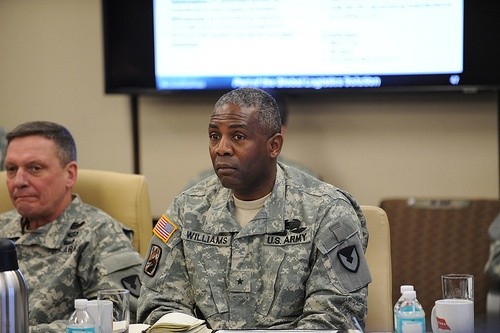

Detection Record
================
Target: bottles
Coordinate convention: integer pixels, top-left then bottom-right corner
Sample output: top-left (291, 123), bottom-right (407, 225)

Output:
top-left (65, 299), bottom-right (95, 333)
top-left (394, 285), bottom-right (426, 333)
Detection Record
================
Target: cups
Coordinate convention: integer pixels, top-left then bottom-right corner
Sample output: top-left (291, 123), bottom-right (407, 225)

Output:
top-left (97, 289), bottom-right (130, 333)
top-left (88, 300), bottom-right (113, 333)
top-left (441, 273), bottom-right (474, 301)
top-left (431, 299), bottom-right (474, 333)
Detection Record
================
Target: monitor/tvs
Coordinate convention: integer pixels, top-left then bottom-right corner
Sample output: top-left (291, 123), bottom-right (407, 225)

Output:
top-left (100, 0), bottom-right (499, 98)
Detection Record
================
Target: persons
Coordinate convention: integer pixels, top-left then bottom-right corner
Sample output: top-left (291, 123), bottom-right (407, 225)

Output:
top-left (0, 121), bottom-right (144, 333)
top-left (137, 87), bottom-right (371, 333)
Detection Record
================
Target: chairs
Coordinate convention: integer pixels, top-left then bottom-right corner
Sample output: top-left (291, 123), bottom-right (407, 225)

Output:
top-left (0, 169), bottom-right (153, 257)
top-left (356, 206), bottom-right (394, 333)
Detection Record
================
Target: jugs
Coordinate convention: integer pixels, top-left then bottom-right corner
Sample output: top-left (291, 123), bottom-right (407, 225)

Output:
top-left (0, 238), bottom-right (30, 333)
